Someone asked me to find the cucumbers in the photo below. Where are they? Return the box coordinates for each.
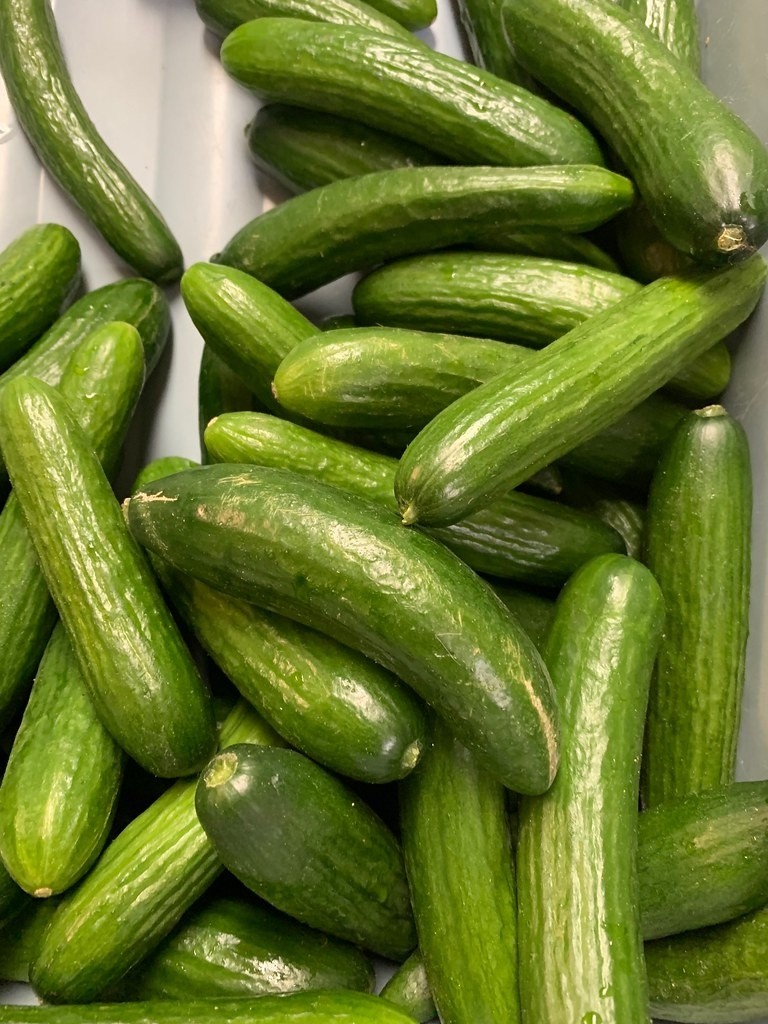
[0,0,768,1024]
